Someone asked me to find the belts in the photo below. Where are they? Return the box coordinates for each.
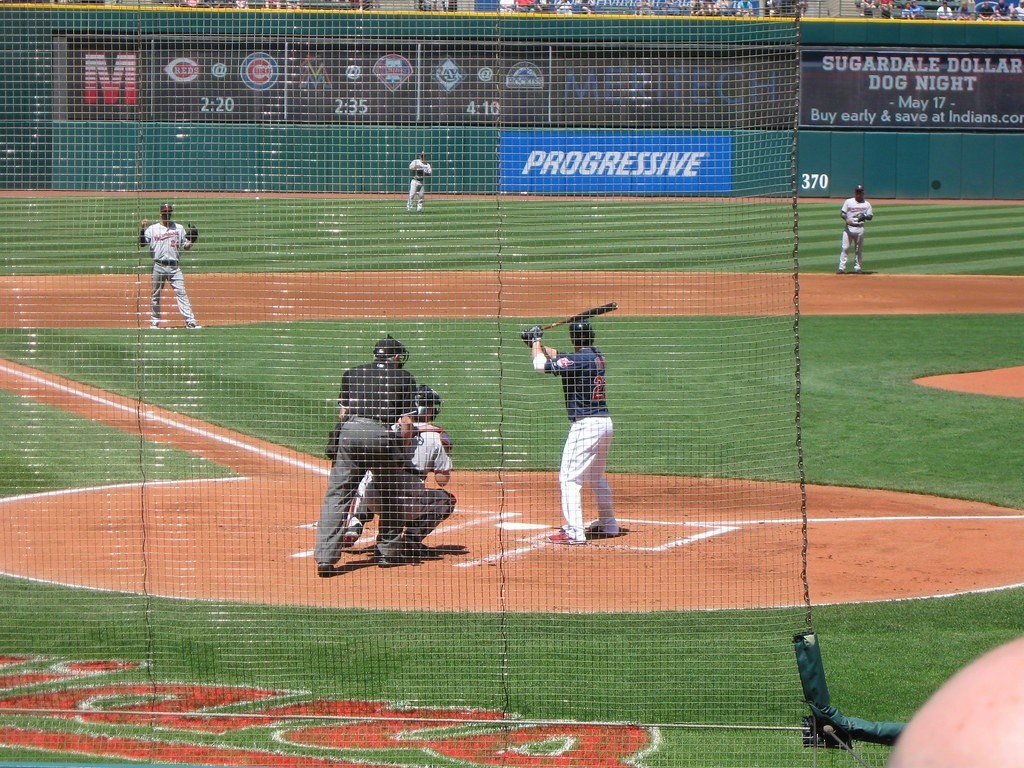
[848,224,859,227]
[348,416,391,428]
[155,260,178,266]
[405,467,423,479]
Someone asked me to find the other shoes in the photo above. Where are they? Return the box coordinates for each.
[378,556,405,568]
[318,561,336,578]
[858,270,861,273]
[549,534,571,545]
[342,524,362,548]
[186,324,202,330]
[588,525,600,533]
[150,325,157,329]
[401,531,431,557]
[836,269,843,274]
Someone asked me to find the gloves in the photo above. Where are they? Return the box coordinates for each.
[525,327,544,347]
[186,224,197,243]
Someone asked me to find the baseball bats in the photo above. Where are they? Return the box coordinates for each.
[521,302,618,338]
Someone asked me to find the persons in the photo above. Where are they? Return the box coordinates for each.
[689,0,755,16]
[419,0,458,12]
[139,203,204,329]
[662,0,681,15]
[766,0,809,18]
[312,334,419,577]
[936,0,1024,21]
[837,185,874,273]
[636,0,655,15]
[175,0,301,10]
[859,0,928,20]
[496,0,597,14]
[528,319,621,545]
[342,381,453,548]
[406,152,432,214]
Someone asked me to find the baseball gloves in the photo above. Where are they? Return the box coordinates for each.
[185,223,198,243]
[854,213,865,222]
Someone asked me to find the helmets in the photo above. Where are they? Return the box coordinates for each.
[415,384,442,419]
[570,320,595,348]
[855,185,864,190]
[420,151,427,156]
[161,203,173,212]
[374,334,409,369]
